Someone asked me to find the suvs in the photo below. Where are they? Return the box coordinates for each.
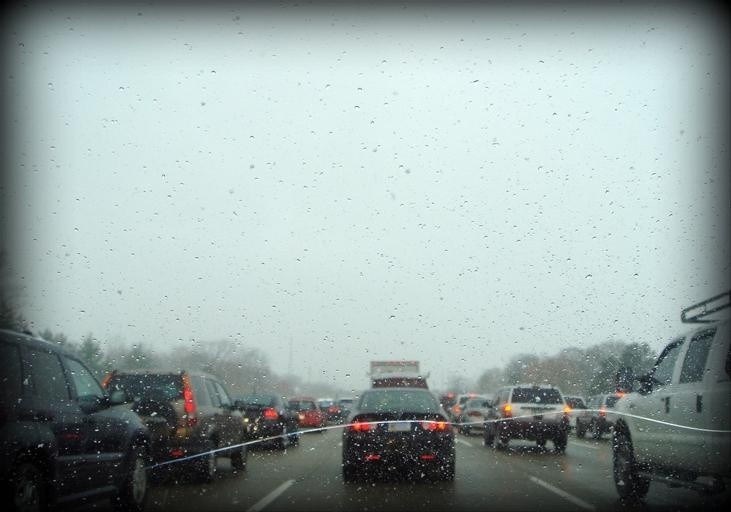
[97,364,253,483]
[0,322,156,510]
[483,382,573,452]
[575,391,629,438]
[608,285,731,500]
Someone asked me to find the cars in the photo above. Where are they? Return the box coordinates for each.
[340,383,459,481]
[287,392,499,435]
[227,389,302,450]
[561,391,586,428]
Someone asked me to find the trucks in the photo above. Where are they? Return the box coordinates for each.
[369,359,428,388]
